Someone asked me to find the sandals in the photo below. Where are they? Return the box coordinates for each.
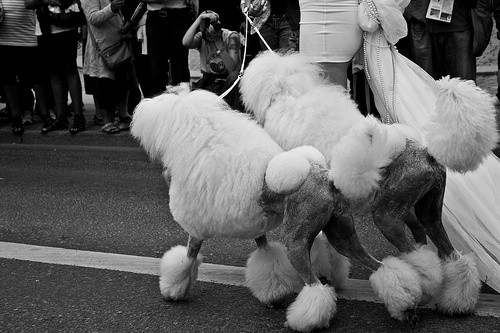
[42,115,69,132]
[70,115,85,134]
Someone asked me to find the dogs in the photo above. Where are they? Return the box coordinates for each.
[239,47,499,319]
[129,83,422,331]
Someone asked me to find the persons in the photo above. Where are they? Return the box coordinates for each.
[0,0,500,137]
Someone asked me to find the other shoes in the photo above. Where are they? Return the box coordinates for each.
[22,110,34,126]
[12,117,24,136]
[101,118,130,133]
[93,114,104,125]
[115,111,132,122]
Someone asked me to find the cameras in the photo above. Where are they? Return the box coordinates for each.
[204,18,221,33]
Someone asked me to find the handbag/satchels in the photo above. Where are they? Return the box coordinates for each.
[101,39,133,70]
